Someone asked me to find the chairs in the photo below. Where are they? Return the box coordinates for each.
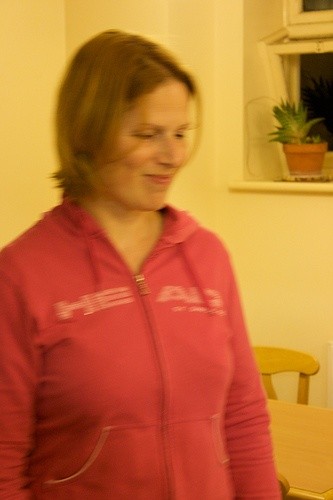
[249,346,320,405]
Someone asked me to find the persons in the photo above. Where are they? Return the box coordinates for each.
[0,29,283,500]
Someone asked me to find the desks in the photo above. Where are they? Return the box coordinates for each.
[266,400,333,500]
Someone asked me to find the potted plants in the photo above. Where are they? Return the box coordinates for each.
[268,98,330,182]
